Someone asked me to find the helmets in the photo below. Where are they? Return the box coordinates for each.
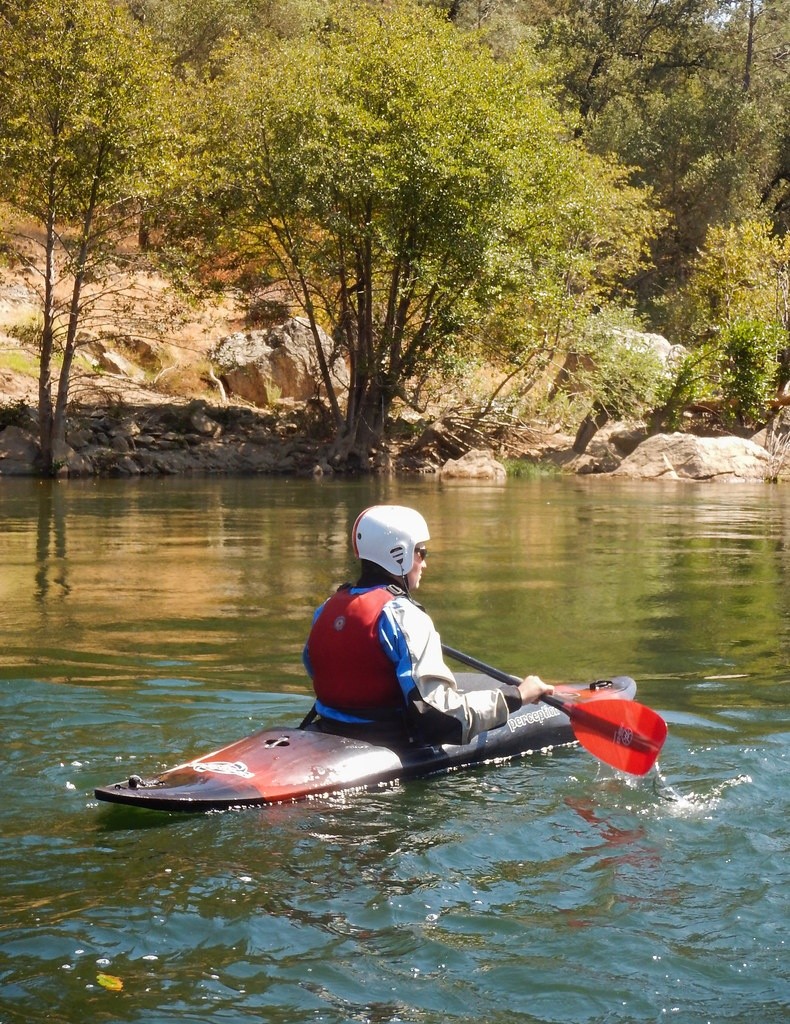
[352,505,430,575]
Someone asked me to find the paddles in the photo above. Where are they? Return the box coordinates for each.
[438,642,670,779]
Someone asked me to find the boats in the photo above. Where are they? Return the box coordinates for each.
[95,678,637,817]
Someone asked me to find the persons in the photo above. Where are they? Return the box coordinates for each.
[303,504,555,752]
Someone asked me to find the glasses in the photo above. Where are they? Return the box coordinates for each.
[414,547,428,562]
